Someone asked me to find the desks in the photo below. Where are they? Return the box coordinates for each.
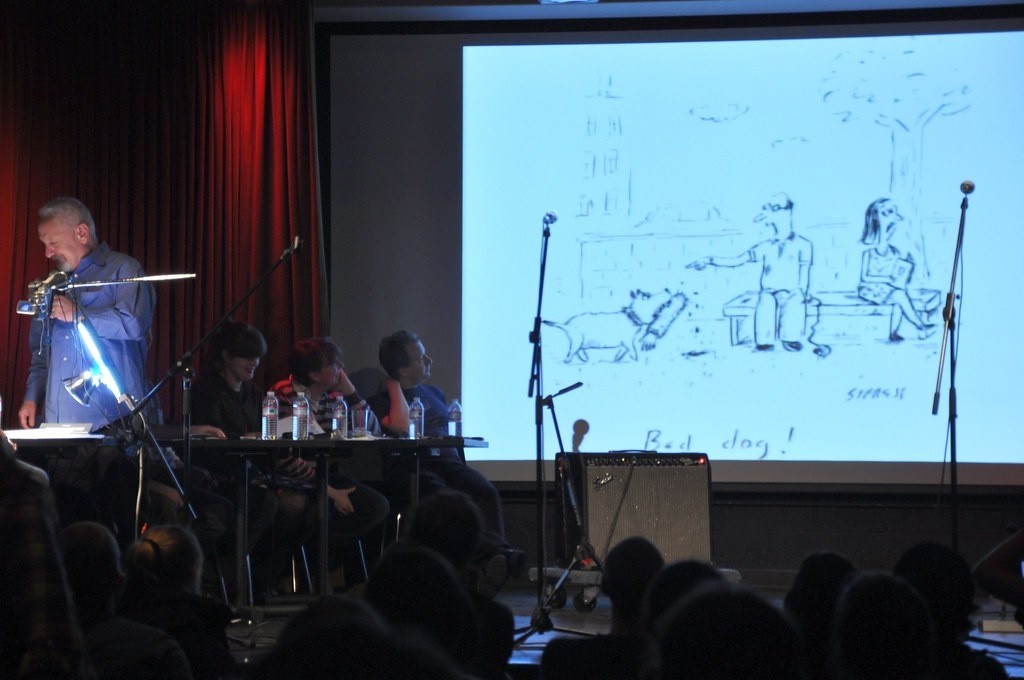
[149,438,489,616]
[9,435,120,527]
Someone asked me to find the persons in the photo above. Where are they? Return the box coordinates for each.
[367,328,529,602]
[17,196,157,567]
[267,334,391,598]
[0,429,517,680]
[540,536,1009,680]
[102,326,237,599]
[991,525,1024,626]
[187,320,314,605]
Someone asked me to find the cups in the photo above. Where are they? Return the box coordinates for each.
[352,410,367,436]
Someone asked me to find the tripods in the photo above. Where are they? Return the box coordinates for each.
[512,223,600,648]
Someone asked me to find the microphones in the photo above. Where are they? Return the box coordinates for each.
[543,210,557,223]
[961,180,975,194]
[47,270,66,297]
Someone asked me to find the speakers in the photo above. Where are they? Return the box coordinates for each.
[555,453,712,565]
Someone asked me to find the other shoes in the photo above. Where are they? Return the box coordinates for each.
[497,547,528,579]
[481,554,507,600]
[249,583,297,603]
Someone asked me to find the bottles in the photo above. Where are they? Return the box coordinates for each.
[261,391,278,439]
[449,398,462,437]
[332,396,347,438]
[409,397,424,440]
[293,392,309,441]
